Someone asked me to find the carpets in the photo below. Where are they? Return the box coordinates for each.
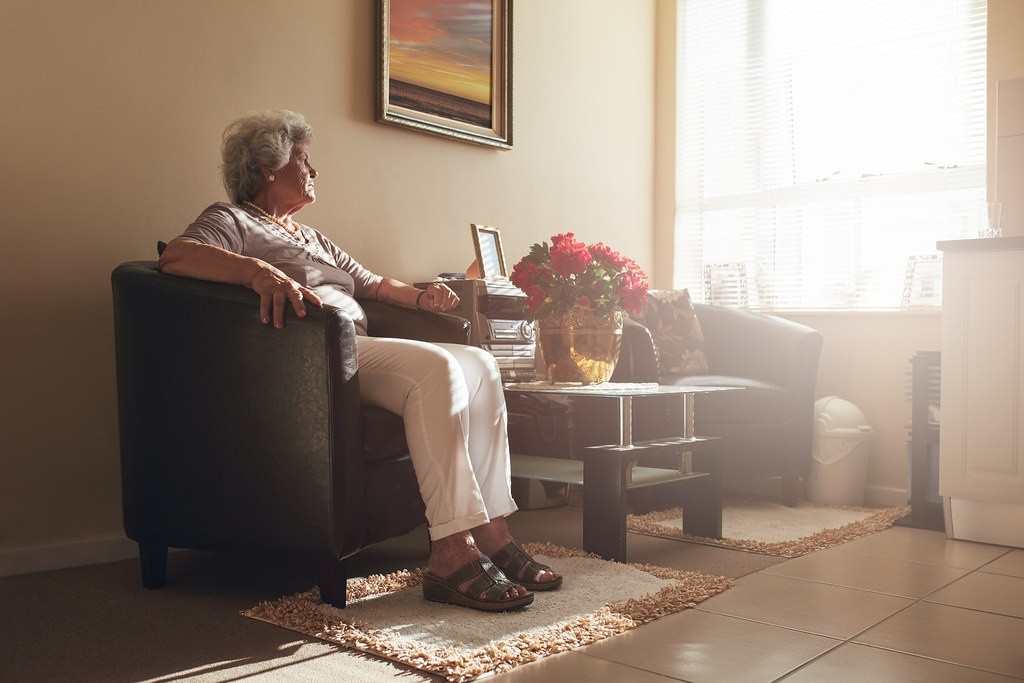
[624,502,912,561]
[240,539,734,683]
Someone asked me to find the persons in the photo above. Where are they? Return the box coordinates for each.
[156,107,565,611]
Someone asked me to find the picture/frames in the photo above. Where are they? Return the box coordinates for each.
[470,224,508,279]
[374,0,514,152]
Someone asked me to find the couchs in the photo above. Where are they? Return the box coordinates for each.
[541,297,824,516]
[110,258,473,608]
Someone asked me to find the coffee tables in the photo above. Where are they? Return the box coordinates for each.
[499,381,745,563]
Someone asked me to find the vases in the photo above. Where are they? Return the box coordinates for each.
[535,311,622,386]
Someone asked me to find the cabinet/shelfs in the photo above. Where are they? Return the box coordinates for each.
[413,278,571,510]
[937,235,1024,549]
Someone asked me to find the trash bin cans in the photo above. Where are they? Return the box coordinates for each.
[806,395,874,507]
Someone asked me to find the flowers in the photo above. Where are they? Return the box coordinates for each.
[510,234,650,313]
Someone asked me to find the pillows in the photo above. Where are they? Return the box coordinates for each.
[630,288,709,376]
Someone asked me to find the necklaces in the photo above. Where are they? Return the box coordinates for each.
[242,199,302,241]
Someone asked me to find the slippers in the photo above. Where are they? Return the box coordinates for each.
[420,558,533,612]
[491,541,562,591]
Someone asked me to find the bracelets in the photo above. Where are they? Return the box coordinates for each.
[416,290,426,311]
